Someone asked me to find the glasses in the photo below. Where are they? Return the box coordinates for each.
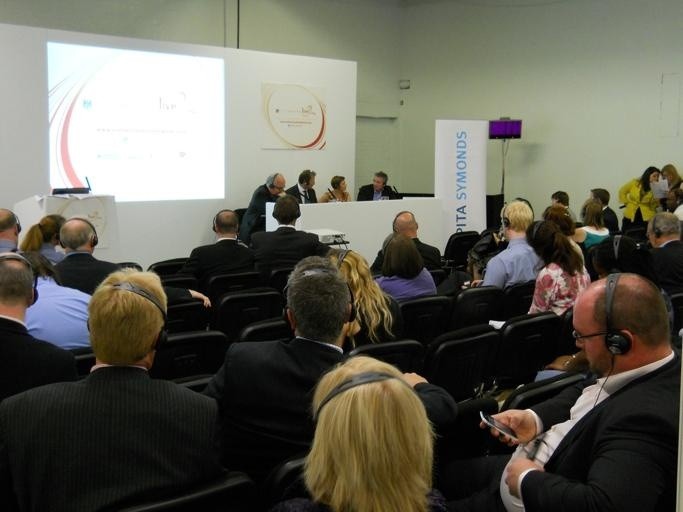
[572,330,606,340]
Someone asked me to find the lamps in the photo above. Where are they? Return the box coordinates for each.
[488,118,521,206]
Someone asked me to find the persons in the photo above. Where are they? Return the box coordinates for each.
[18,250,93,351]
[373,232,437,301]
[665,189,683,221]
[284,170,318,204]
[570,199,609,250]
[268,355,448,512]
[51,218,121,296]
[436,199,545,297]
[544,235,674,371]
[658,164,683,213]
[0,251,80,403]
[238,173,286,248]
[248,196,332,270]
[589,189,619,232]
[645,212,683,296]
[19,215,67,266]
[369,211,442,276]
[0,209,24,255]
[318,175,351,203]
[428,273,682,511]
[0,267,220,512]
[198,256,458,508]
[542,205,584,267]
[525,220,591,317]
[356,171,402,201]
[189,210,255,291]
[551,191,576,224]
[324,248,401,361]
[618,166,660,235]
[450,198,534,282]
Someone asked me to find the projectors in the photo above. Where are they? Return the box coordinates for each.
[303,228,345,245]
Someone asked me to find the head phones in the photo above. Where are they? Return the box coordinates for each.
[303,170,313,186]
[392,210,419,232]
[281,267,358,325]
[269,172,279,189]
[58,218,100,249]
[606,234,623,273]
[0,251,39,308]
[604,273,631,356]
[85,281,170,352]
[212,209,241,235]
[653,214,662,239]
[310,370,438,429]
[530,221,547,247]
[500,198,535,227]
[15,212,22,235]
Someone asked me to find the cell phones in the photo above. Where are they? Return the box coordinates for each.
[479,410,519,442]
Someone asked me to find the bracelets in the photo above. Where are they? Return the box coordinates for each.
[559,354,578,368]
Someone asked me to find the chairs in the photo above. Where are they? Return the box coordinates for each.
[582,242,606,280]
[217,289,283,331]
[429,326,502,397]
[500,313,560,370]
[235,315,292,342]
[207,274,262,296]
[166,302,214,333]
[266,269,294,291]
[426,269,446,288]
[167,330,227,371]
[123,475,257,512]
[162,276,201,296]
[263,452,310,512]
[503,281,535,315]
[455,395,499,420]
[348,341,426,374]
[149,258,190,276]
[397,296,453,339]
[625,228,649,244]
[234,208,249,232]
[446,231,479,263]
[502,373,585,413]
[452,285,502,329]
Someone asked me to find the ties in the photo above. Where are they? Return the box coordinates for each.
[304,190,309,203]
